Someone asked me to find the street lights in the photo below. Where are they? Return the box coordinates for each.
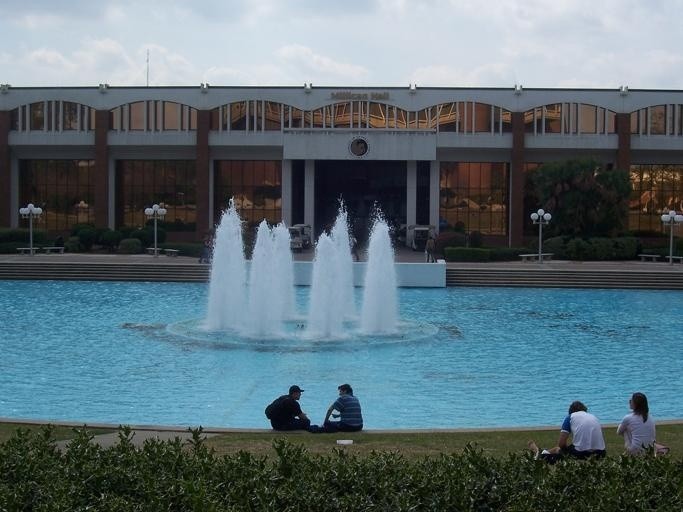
[19,203,43,257]
[530,208,552,264]
[661,210,683,266]
[144,204,168,259]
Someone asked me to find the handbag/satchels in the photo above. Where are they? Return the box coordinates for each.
[266,399,282,419]
[541,453,559,465]
[654,443,670,456]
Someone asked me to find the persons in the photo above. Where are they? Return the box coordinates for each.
[528,400,608,461]
[615,392,656,456]
[349,231,360,261]
[270,385,311,431]
[198,236,213,264]
[321,383,364,432]
[425,236,436,263]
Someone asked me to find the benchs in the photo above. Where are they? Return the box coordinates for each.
[145,247,180,257]
[637,252,683,265]
[517,252,556,264]
[16,247,66,255]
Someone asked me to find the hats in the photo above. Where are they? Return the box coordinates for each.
[289,385,304,393]
